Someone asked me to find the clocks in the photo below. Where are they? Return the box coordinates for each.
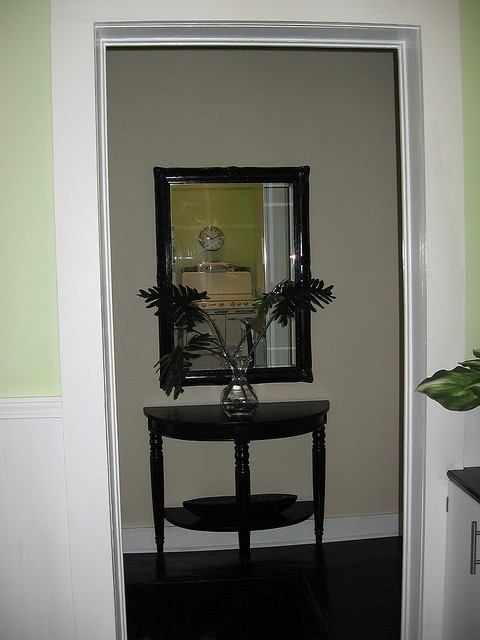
[199,226,225,251]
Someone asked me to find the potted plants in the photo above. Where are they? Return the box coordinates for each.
[137,273,336,421]
[169,275,299,370]
[414,347,479,412]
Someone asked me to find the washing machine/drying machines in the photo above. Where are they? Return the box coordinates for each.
[181,259,256,372]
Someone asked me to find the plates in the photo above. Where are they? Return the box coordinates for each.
[183,494,298,518]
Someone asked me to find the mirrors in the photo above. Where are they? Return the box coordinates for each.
[154,165,314,388]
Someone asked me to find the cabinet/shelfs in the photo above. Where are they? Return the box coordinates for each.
[140,399,332,573]
[444,465,480,640]
